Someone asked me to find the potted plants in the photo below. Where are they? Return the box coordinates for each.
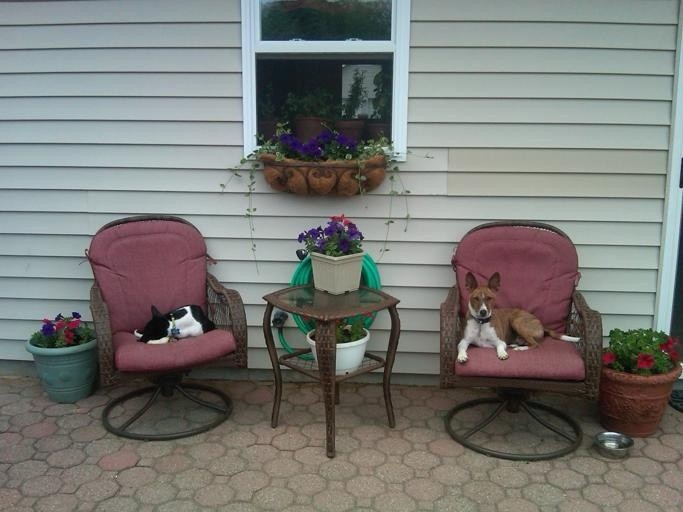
[256,85,284,140]
[289,79,328,140]
[370,76,394,144]
[337,70,363,144]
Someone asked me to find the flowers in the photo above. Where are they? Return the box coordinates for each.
[309,315,375,344]
[35,308,87,342]
[599,324,681,376]
[25,310,95,351]
[293,214,366,261]
[296,214,365,253]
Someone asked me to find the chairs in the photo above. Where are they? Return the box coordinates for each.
[439,219,603,461]
[82,211,249,441]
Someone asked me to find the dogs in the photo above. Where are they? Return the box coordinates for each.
[457,270,581,367]
[133,301,217,347]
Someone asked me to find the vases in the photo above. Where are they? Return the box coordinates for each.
[306,323,370,373]
[22,327,97,403]
[304,249,368,296]
[591,359,682,437]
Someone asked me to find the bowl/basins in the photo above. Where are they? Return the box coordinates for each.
[596,432,634,459]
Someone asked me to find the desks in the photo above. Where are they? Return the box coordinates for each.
[271,283,403,458]
